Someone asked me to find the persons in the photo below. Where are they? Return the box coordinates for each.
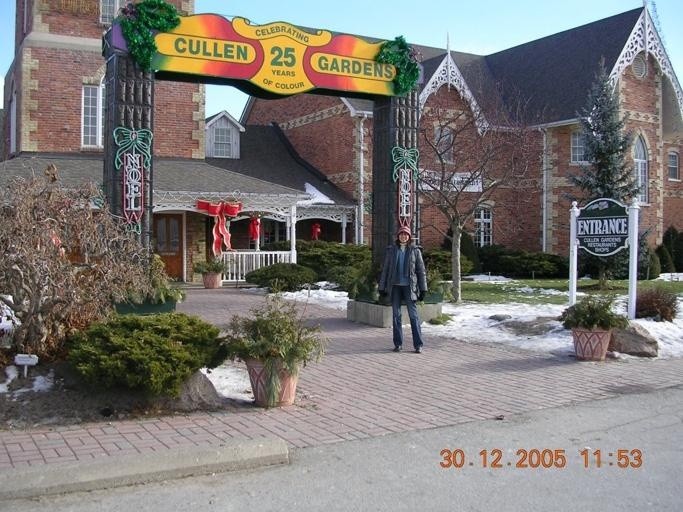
[377,225,430,354]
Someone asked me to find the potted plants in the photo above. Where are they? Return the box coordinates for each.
[222,278,332,407]
[191,254,231,288]
[556,293,632,361]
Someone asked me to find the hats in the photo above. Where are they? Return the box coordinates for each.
[396,227,411,237]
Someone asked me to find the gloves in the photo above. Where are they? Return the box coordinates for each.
[379,290,387,297]
[418,291,425,301]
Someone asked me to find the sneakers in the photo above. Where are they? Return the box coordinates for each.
[394,345,402,352]
[415,347,422,353]
